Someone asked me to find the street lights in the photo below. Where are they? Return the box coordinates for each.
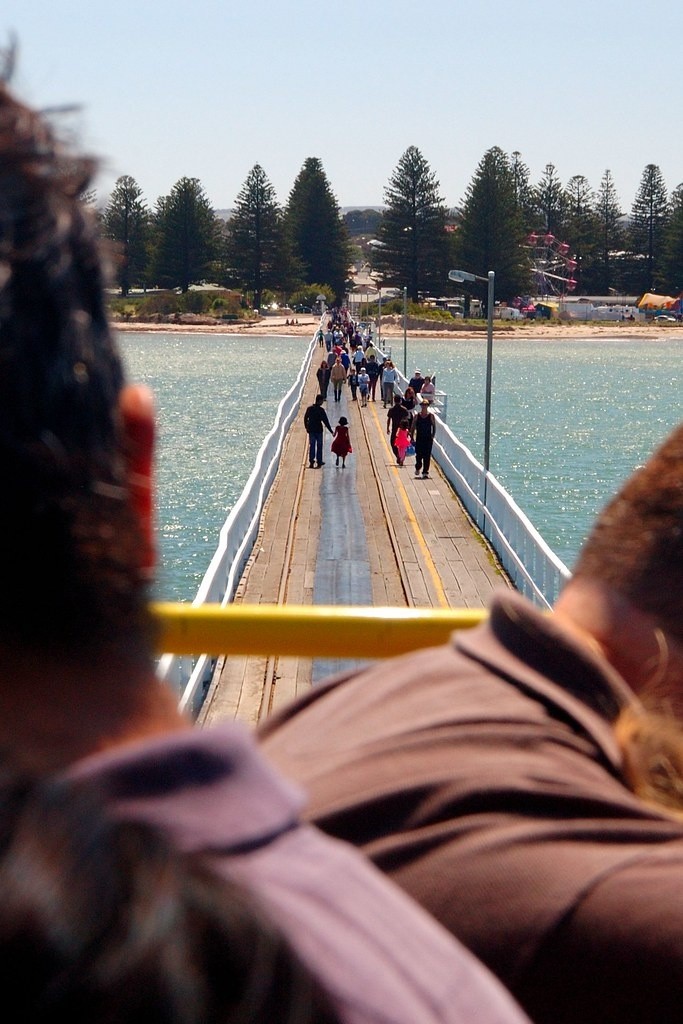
[447,268,495,472]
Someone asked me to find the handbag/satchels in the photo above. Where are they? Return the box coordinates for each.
[353,358,355,365]
[406,445,415,457]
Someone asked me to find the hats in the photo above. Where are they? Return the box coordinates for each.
[335,357,342,362]
[415,368,421,373]
[361,367,366,372]
[419,399,429,406]
[340,350,346,354]
[338,417,348,425]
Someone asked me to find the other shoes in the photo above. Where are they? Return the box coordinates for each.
[372,398,376,402]
[317,461,325,465]
[361,403,367,408]
[424,472,429,478]
[336,460,339,466]
[310,459,314,463]
[415,470,420,475]
[342,464,346,469]
[367,395,369,400]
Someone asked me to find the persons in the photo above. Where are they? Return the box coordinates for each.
[0,57,532,1024]
[253,428,683,1024]
[304,306,437,478]
